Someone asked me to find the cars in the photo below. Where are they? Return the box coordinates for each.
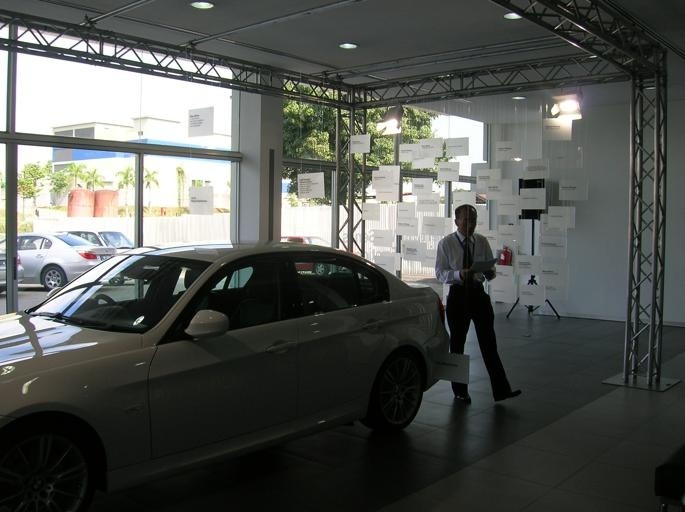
[1,242,453,510]
[281,235,358,276]
[0,228,136,294]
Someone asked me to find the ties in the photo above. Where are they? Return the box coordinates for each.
[462,238,475,282]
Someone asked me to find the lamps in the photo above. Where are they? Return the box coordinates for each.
[558,85,584,121]
[377,105,404,134]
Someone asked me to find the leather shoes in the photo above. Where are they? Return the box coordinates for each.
[494,390,521,401]
[456,394,472,403]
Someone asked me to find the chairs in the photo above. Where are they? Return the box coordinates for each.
[324,271,359,307]
[145,269,216,336]
[229,278,277,330]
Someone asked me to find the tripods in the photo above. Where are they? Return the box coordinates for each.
[503,220,561,320]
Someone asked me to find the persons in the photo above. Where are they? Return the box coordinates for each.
[435,205,522,404]
